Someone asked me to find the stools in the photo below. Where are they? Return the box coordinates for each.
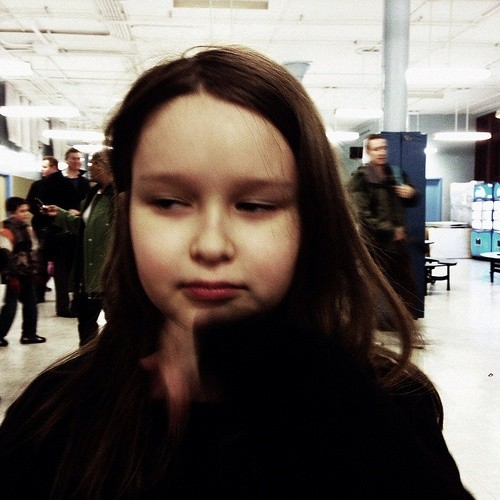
[480,252,500,283]
[425,256,457,291]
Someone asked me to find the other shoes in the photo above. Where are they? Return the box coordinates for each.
[45,286,51,292]
[20,335,46,344]
[0,337,9,348]
[56,309,77,318]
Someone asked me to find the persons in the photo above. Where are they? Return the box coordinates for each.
[0,47,475,500]
[25,149,115,347]
[0,197,46,347]
[349,135,430,348]
